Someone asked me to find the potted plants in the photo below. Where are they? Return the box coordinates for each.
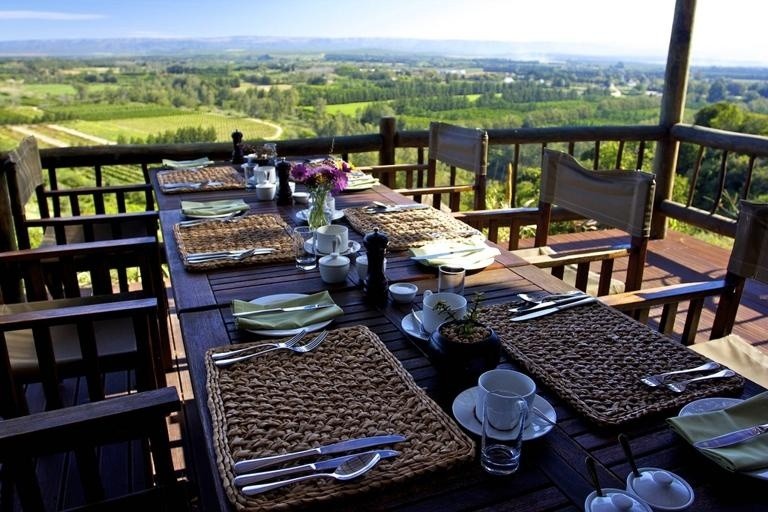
[426,291,500,378]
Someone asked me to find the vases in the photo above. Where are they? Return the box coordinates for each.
[307,195,334,246]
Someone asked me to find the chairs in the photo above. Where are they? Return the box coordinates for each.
[1,297,185,512]
[452,149,655,296]
[589,200,768,391]
[3,236,175,391]
[1,134,160,297]
[355,122,490,214]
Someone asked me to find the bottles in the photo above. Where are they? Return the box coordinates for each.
[363,227,389,296]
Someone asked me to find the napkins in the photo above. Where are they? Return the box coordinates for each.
[668,392,768,471]
[230,288,344,331]
[176,198,251,220]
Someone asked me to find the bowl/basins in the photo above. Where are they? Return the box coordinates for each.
[388,283,417,303]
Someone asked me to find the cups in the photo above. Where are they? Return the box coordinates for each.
[583,487,654,512]
[478,391,524,477]
[315,252,389,282]
[476,368,538,430]
[625,466,697,511]
[423,263,467,333]
[308,197,335,216]
[264,143,278,162]
[316,224,348,250]
[292,226,318,272]
[240,161,336,215]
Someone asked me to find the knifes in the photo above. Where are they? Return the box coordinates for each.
[235,433,406,472]
[235,302,333,320]
[187,248,277,258]
[365,203,428,212]
[232,448,401,488]
[507,293,596,323]
[692,423,768,453]
[409,245,488,261]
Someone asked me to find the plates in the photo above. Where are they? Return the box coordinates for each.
[244,293,335,336]
[448,382,561,438]
[294,209,348,223]
[399,310,430,342]
[342,182,374,191]
[675,396,743,420]
[302,237,360,255]
[413,246,501,271]
[183,211,244,219]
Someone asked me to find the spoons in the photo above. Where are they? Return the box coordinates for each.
[240,450,381,499]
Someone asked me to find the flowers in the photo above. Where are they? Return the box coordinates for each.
[287,158,354,195]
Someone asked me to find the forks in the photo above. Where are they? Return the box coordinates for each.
[206,326,330,365]
[187,249,256,265]
[631,361,736,392]
